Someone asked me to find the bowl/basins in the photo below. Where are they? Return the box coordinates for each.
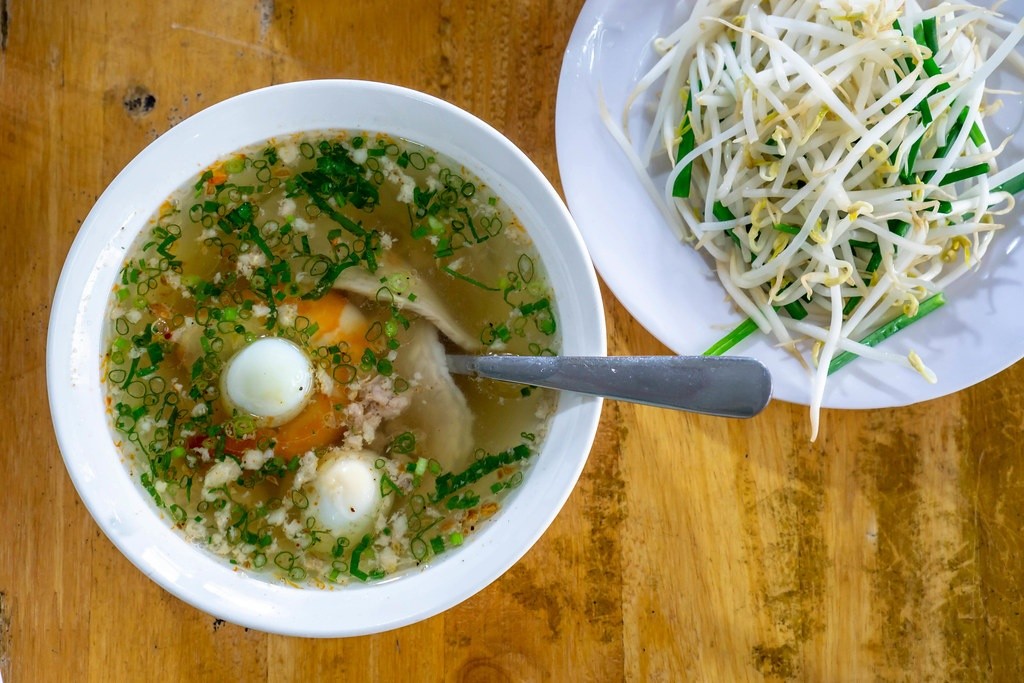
[42,78,614,640]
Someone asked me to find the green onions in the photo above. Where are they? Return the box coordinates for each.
[107,135,558,587]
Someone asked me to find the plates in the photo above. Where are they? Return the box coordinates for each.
[552,0,1024,409]
